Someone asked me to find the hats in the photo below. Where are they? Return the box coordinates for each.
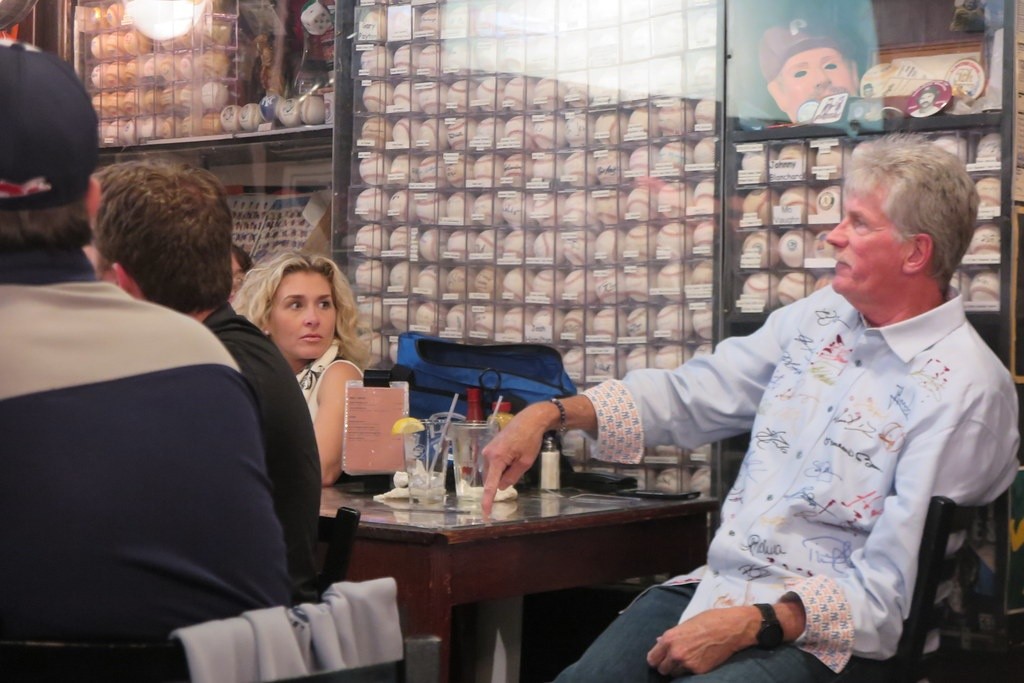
[0,42,98,210]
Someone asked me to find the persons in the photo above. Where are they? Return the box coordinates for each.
[0,35,292,643]
[480,133,1021,683]
[232,247,373,486]
[229,242,253,301]
[94,159,321,606]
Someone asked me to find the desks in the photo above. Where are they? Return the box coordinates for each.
[309,489,721,683]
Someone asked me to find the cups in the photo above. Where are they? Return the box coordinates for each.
[447,422,499,509]
[402,419,452,506]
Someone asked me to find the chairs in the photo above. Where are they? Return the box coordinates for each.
[883,496,986,683]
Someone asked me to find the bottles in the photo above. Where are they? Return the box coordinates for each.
[486,402,516,432]
[539,437,561,493]
[459,388,488,489]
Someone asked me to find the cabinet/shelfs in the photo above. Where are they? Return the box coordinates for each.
[63,1,1023,626]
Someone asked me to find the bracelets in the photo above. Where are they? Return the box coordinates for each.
[549,398,566,432]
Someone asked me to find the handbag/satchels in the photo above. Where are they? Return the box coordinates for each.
[395,332,577,475]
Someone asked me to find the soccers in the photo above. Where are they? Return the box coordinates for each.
[350,1,710,499]
[87,5,336,140]
[733,131,1001,306]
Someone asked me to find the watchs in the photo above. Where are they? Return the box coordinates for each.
[754,603,783,649]
